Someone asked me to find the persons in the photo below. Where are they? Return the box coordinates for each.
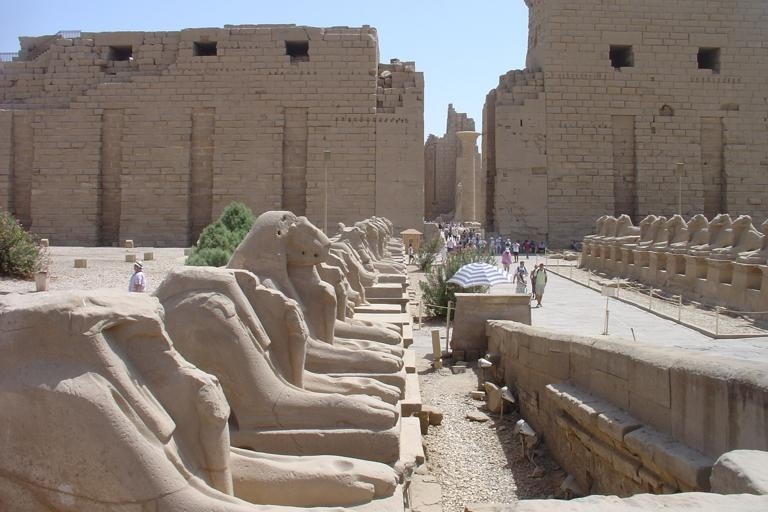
[129,260,146,292]
[407,218,580,308]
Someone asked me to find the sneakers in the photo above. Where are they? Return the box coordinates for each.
[536,304,543,307]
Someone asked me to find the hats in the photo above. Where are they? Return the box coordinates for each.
[538,264,545,266]
[133,261,143,268]
